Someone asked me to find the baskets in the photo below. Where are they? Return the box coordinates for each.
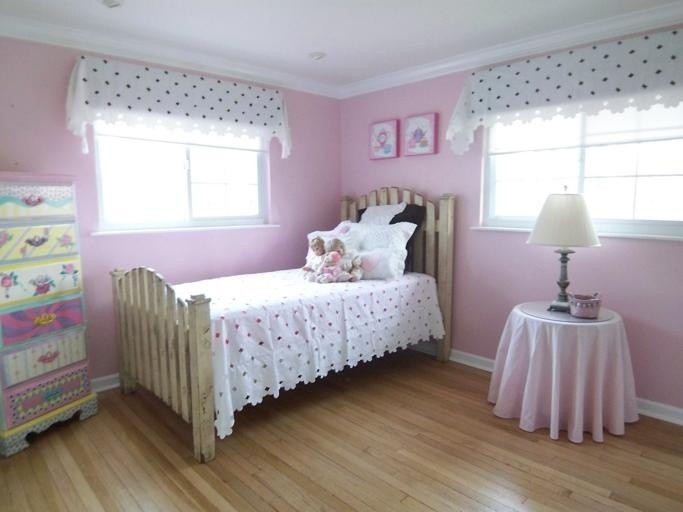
[566,292,602,319]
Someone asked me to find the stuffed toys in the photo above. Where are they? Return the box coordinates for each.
[316,251,350,283]
[301,237,325,281]
[314,239,363,281]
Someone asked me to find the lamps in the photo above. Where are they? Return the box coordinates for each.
[526,190,600,314]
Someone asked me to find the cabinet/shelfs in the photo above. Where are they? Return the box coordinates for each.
[0,171,100,456]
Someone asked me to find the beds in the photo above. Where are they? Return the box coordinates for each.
[108,189,457,464]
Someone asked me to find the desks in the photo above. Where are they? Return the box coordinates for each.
[483,301,641,447]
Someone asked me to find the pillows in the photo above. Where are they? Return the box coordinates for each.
[334,220,416,281]
[355,202,424,248]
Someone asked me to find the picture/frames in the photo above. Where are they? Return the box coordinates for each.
[366,119,399,161]
[400,112,440,157]
